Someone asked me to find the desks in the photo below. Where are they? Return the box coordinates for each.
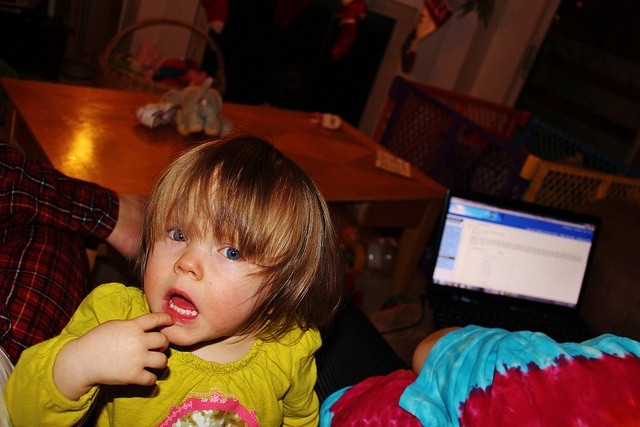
[1,72,451,320]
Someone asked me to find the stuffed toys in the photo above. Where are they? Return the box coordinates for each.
[133,76,234,137]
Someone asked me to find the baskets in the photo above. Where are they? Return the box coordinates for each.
[98,15,226,101]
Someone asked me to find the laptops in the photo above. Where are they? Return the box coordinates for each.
[429,190,602,342]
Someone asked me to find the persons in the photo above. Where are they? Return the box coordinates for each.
[3,133,345,426]
[1,142,150,368]
[314,322,640,426]
